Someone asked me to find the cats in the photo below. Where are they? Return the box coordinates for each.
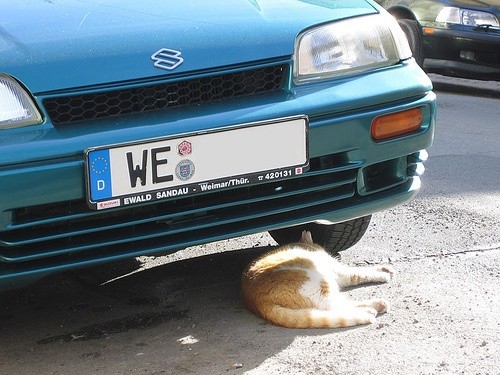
[241,230,394,329]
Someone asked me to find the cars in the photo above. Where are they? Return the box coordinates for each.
[0,0,436,299]
[383,0,500,82]
